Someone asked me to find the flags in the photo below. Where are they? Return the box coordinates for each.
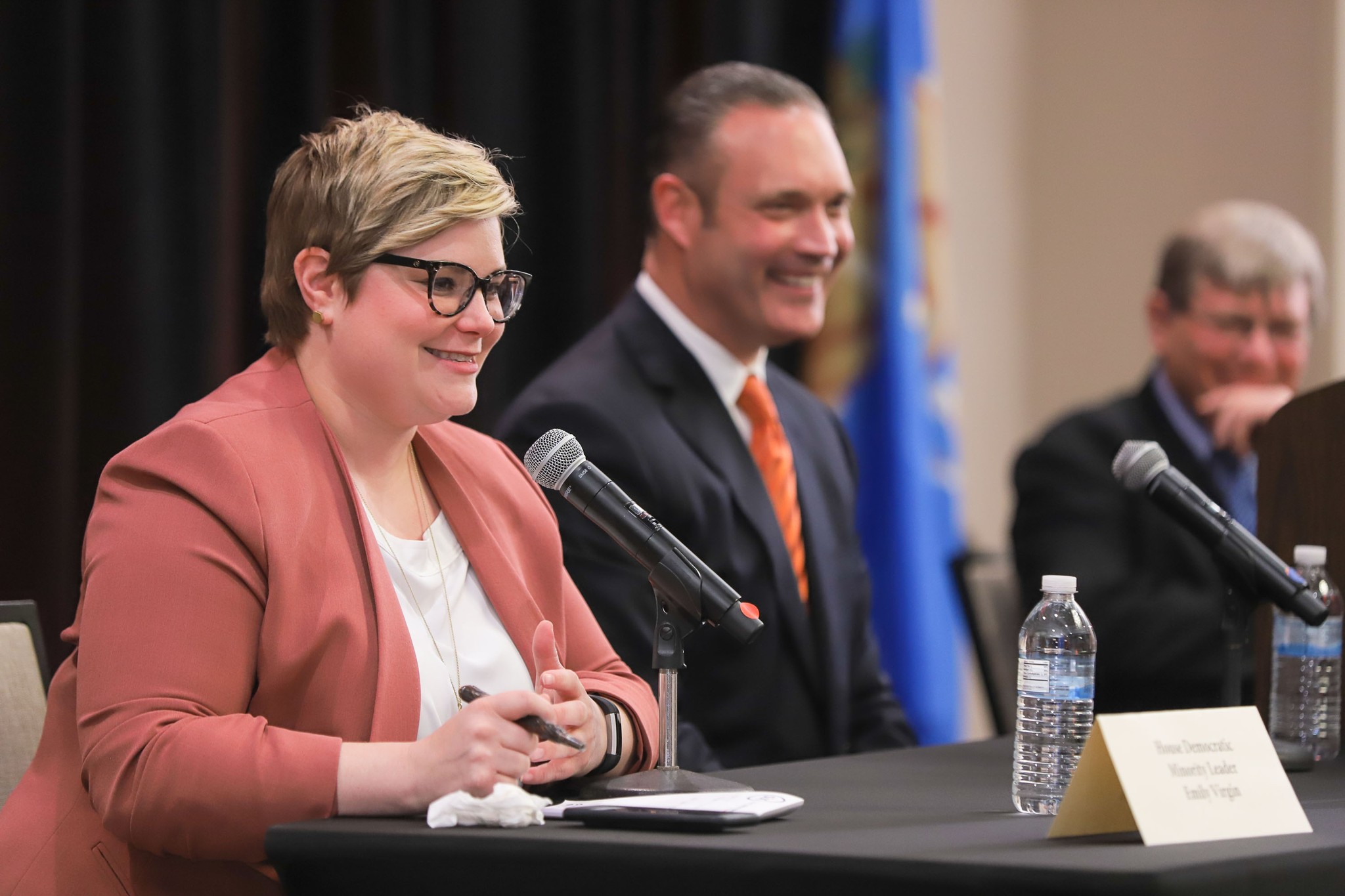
[791,0,1005,743]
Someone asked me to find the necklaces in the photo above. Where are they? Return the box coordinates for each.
[356,441,462,713]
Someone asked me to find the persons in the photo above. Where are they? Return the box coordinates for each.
[1008,195,1324,723]
[0,104,661,896]
[493,59,913,772]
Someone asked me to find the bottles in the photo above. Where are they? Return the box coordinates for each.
[1268,545,1344,763]
[1012,575,1097,814]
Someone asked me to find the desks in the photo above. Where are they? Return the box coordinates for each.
[261,728,1345,896]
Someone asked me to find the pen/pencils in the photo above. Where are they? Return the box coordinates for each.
[457,684,586,750]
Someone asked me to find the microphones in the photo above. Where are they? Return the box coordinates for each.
[1113,439,1329,627]
[523,428,766,644]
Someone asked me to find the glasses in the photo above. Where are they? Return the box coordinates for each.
[366,254,533,323]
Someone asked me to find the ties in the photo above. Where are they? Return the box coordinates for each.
[737,373,807,611]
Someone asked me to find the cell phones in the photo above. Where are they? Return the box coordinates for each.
[563,806,757,831]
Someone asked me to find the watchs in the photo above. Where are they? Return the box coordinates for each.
[581,694,622,778]
